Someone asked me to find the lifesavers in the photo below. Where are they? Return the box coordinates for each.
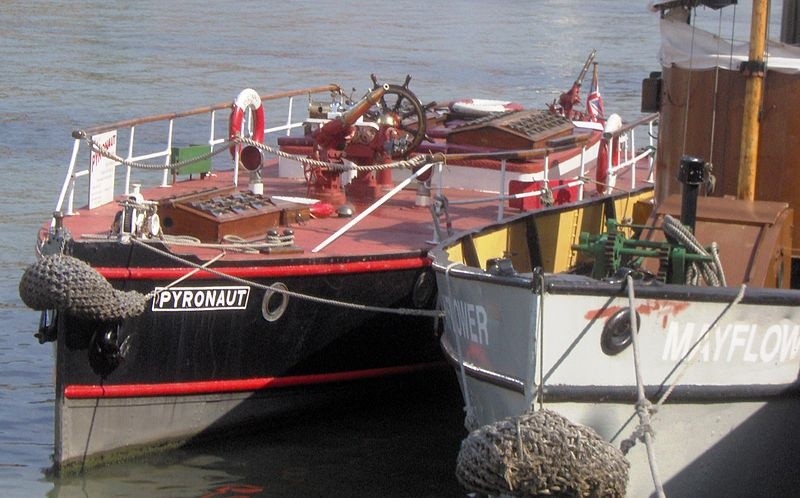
[448,97,521,119]
[229,87,265,165]
[595,112,625,196]
[271,194,335,219]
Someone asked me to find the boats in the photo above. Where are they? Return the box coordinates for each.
[425,2,800,498]
[28,47,657,479]
